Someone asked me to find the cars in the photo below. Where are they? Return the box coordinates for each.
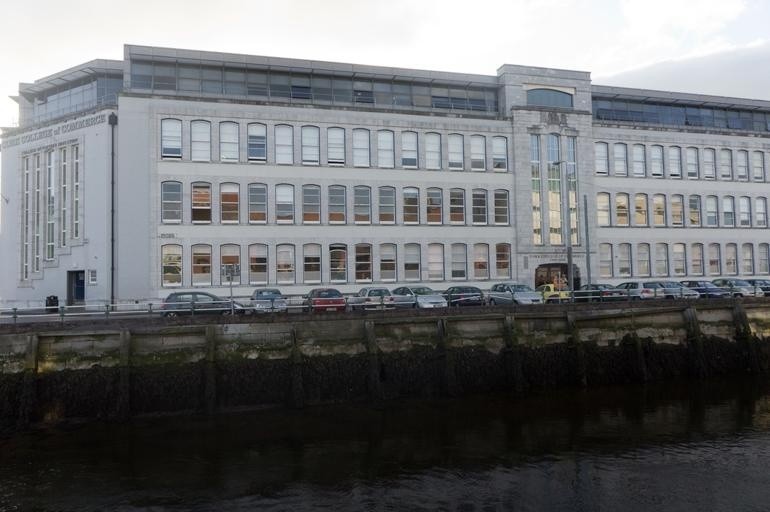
[248,287,289,312]
[351,285,393,311]
[161,265,182,283]
[302,288,346,312]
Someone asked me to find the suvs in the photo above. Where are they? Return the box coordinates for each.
[159,291,246,319]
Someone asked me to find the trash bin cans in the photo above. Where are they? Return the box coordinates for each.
[46,295,58,314]
